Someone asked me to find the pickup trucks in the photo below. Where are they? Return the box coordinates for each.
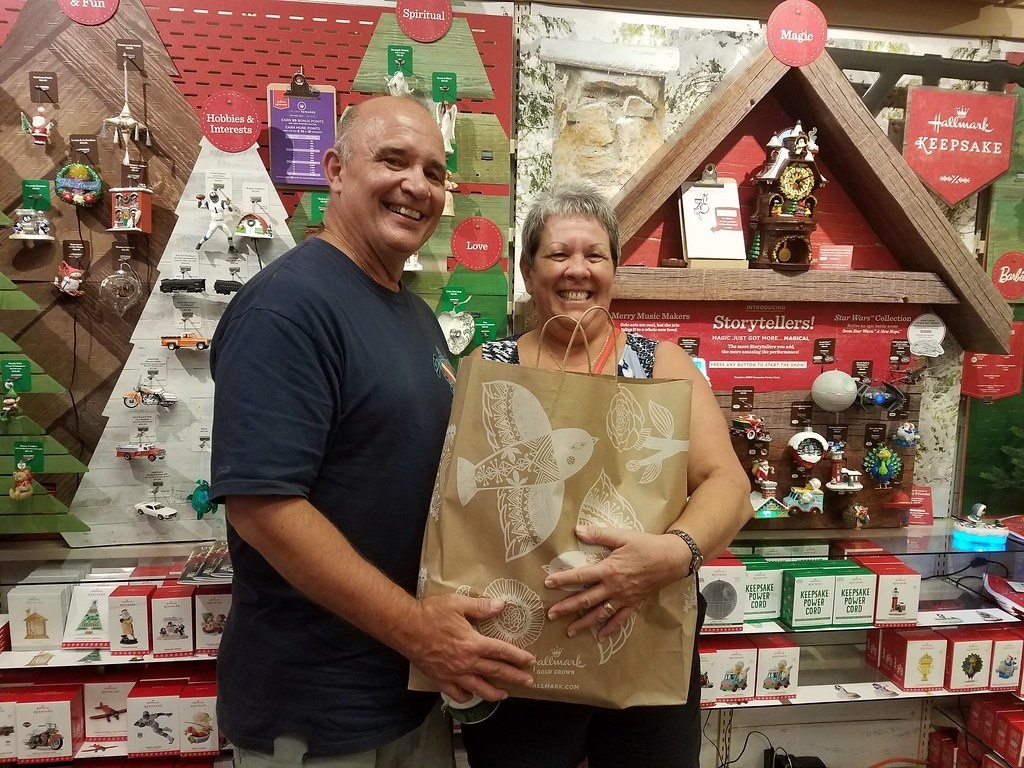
[115,441,166,462]
[161,331,212,351]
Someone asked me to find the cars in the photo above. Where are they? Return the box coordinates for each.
[134,501,178,520]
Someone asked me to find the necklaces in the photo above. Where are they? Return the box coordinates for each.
[539,327,610,371]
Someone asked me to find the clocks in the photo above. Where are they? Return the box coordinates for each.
[750,120,828,271]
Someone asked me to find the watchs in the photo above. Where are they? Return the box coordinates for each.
[666,529,704,578]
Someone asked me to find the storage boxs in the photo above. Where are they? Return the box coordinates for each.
[0,563,238,764]
[698,541,1024,768]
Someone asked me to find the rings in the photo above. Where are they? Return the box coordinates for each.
[602,600,617,616]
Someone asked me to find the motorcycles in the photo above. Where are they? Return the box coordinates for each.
[122,376,177,408]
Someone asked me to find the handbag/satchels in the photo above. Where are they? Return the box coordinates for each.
[405,304,702,711]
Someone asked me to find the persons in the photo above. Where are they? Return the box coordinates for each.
[463,182,753,768]
[133,711,175,746]
[208,92,538,768]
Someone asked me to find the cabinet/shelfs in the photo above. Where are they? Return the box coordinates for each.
[0,579,235,768]
[701,537,1024,711]
